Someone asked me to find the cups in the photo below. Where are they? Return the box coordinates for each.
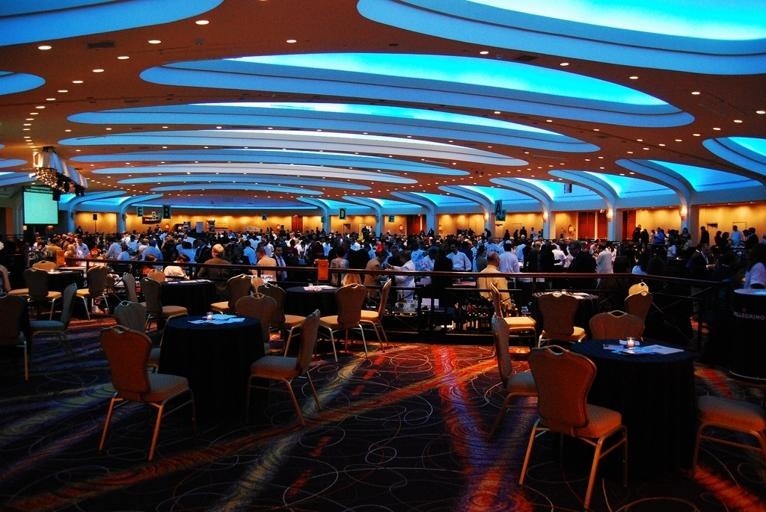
[627,336,637,350]
[205,311,213,320]
[561,288,567,294]
[103,307,109,314]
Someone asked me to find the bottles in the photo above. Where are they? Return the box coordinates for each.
[396,292,418,312]
[745,270,752,291]
[451,300,530,330]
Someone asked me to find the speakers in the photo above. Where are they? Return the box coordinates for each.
[93,214,97,220]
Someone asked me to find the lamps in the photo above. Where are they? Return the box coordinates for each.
[34,146,89,197]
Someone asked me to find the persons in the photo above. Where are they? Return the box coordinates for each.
[1,224,766,318]
